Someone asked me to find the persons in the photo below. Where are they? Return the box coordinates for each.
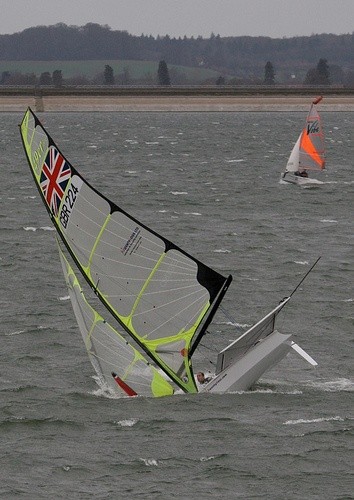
[300,169,309,177]
[196,372,213,384]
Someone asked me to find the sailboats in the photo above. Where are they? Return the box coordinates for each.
[279,95,330,188]
[19,105,319,398]
